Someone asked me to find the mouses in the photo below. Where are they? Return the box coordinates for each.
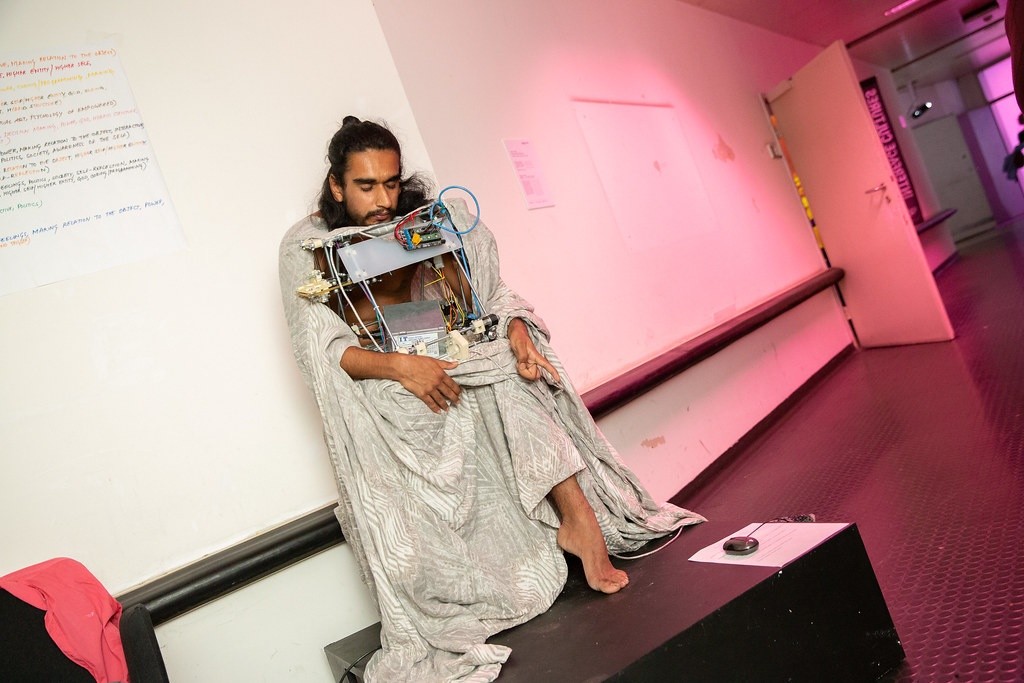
[723,537,759,556]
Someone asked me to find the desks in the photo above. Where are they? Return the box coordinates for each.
[326,523,912,683]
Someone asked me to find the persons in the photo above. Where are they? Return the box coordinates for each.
[275,112,708,683]
